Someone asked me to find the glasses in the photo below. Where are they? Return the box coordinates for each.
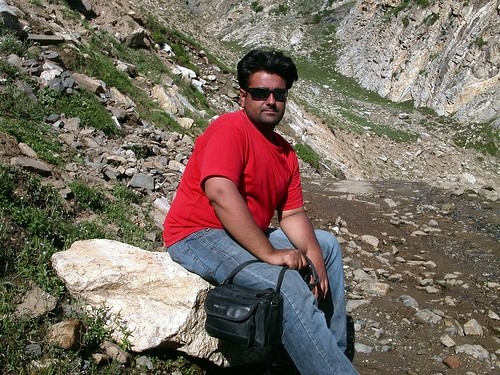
[242,87,289,103]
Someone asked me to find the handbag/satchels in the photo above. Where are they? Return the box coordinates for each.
[203,284,283,354]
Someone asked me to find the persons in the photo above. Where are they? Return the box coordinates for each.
[163,49,358,375]
